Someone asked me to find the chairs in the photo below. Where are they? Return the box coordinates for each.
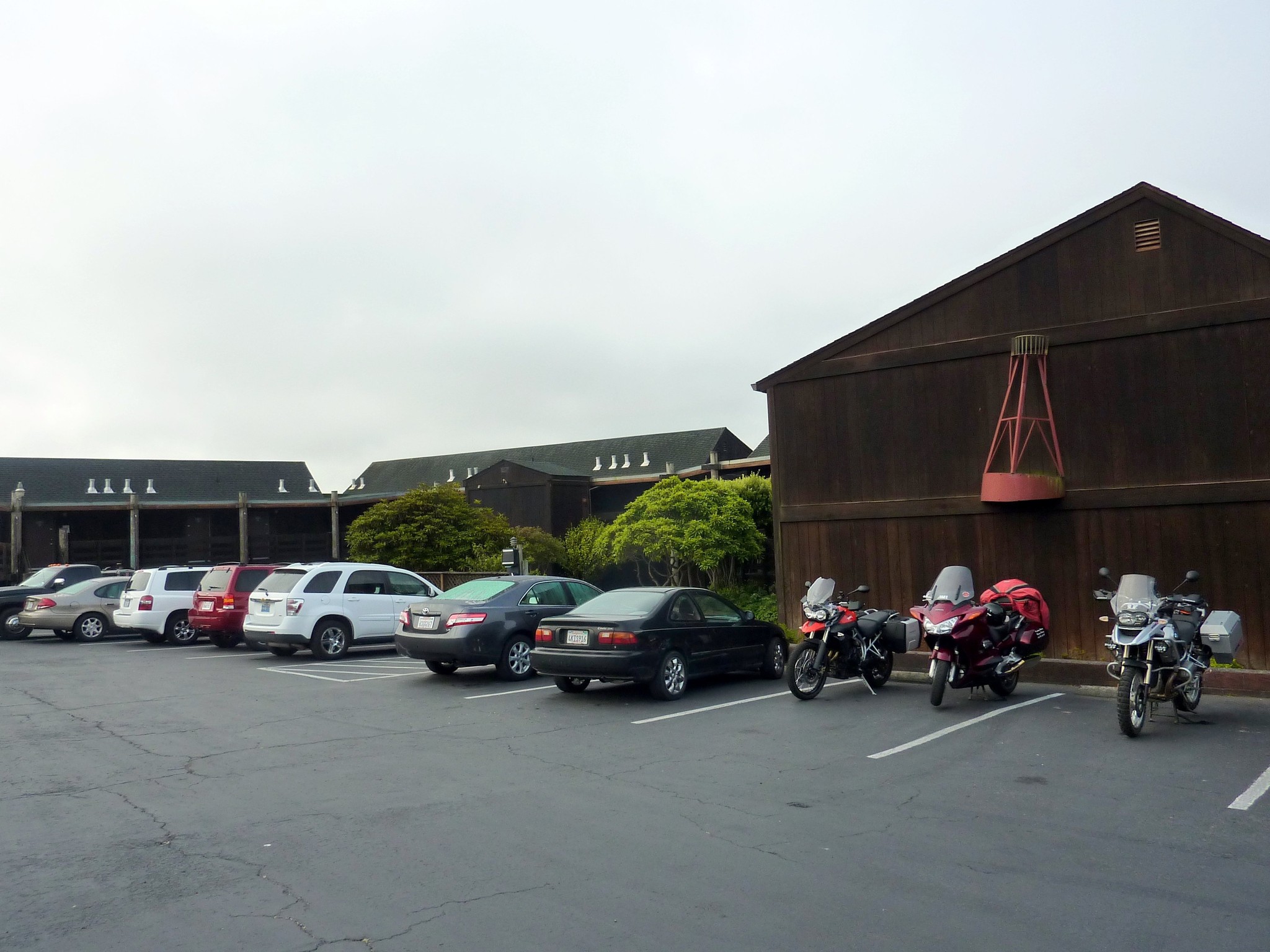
[349,576,366,593]
[679,600,695,621]
[367,575,384,594]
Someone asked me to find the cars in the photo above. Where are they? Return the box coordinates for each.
[528,586,790,699]
[17,575,138,639]
[393,576,608,681]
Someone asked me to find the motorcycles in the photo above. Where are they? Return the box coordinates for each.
[785,577,924,699]
[908,566,1051,705]
[1090,566,1243,733]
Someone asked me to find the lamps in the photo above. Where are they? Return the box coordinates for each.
[510,537,518,550]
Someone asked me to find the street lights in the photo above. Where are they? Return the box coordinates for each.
[9,481,26,585]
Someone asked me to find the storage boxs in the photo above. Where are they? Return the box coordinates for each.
[1200,610,1244,664]
[1015,621,1050,657]
[885,616,923,654]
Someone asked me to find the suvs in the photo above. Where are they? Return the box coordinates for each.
[187,561,293,651]
[244,561,446,660]
[0,564,102,638]
[101,567,137,577]
[112,566,222,646]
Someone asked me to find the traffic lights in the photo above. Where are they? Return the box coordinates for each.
[502,548,516,566]
